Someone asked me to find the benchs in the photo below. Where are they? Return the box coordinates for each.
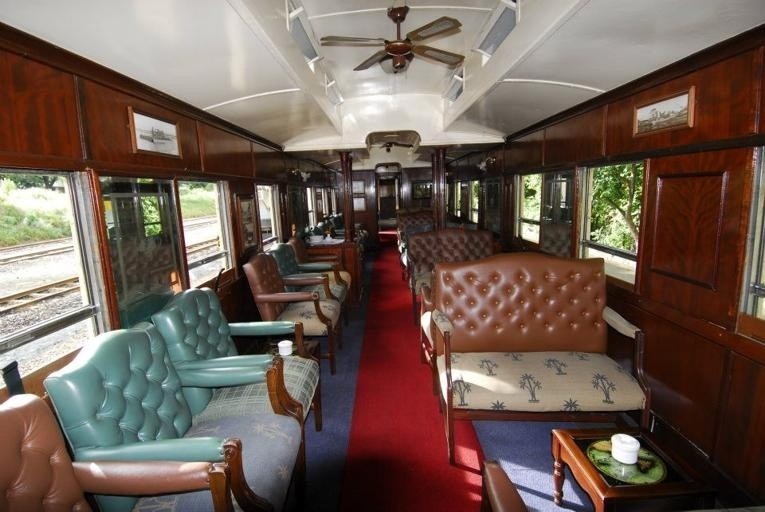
[396,207,499,326]
[419,251,651,466]
[480,462,529,511]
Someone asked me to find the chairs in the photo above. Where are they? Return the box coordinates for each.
[242,236,353,376]
[149,286,323,476]
[307,213,369,264]
[1,321,323,511]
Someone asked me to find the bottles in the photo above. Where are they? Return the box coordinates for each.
[610,432,641,466]
[276,338,294,356]
[325,233,333,243]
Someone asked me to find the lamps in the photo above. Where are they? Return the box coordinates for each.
[474,154,496,174]
[379,54,414,74]
[291,168,313,185]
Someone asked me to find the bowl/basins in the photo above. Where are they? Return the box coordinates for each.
[309,235,322,243]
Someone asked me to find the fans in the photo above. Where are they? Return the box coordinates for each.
[319,6,465,75]
[378,142,413,153]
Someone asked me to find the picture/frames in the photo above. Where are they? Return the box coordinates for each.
[352,179,366,195]
[352,197,368,213]
[231,192,262,262]
[411,179,433,200]
[631,83,698,141]
[126,102,185,163]
